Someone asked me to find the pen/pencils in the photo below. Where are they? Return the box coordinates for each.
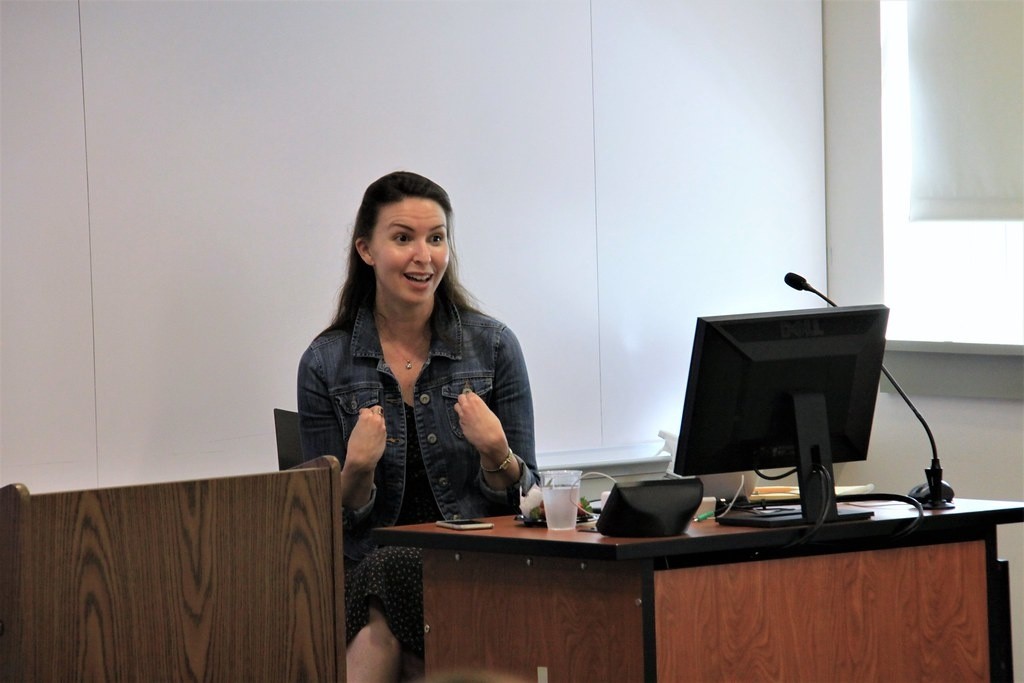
[693,511,715,523]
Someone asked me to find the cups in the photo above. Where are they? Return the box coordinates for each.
[538,470,583,531]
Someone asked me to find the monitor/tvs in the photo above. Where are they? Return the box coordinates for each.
[671,304,890,528]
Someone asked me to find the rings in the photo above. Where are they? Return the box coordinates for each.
[374,407,384,415]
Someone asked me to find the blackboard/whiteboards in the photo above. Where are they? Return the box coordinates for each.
[0,1,830,497]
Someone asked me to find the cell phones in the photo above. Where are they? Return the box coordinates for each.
[436,518,494,530]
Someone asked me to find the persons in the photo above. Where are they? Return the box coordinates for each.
[297,171,541,683]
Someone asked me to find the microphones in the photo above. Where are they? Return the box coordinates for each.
[784,273,956,510]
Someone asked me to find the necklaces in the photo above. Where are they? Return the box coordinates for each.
[381,328,427,370]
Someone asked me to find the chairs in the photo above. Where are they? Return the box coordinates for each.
[0,456,346,683]
[271,404,305,472]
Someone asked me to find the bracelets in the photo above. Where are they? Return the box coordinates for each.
[480,449,512,472]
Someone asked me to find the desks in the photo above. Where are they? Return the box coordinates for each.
[371,501,1024,683]
